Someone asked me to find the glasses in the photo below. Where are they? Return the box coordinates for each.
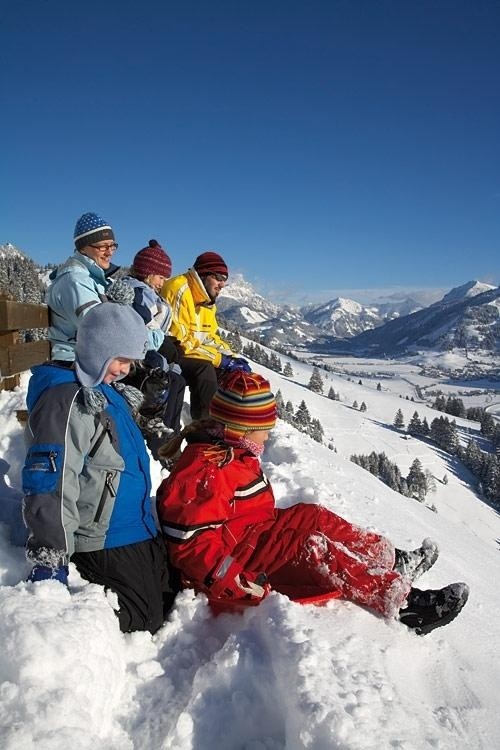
[89,244,118,251]
[211,272,228,283]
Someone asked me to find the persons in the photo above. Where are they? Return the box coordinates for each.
[157,370,469,637]
[47,213,181,440]
[160,251,252,419]
[22,280,171,635]
[122,240,185,471]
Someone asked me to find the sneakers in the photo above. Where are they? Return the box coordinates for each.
[135,412,175,439]
[399,583,470,636]
[160,451,183,472]
[393,537,439,582]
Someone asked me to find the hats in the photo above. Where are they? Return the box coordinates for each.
[74,212,115,252]
[133,240,172,284]
[193,252,229,276]
[204,371,278,466]
[73,301,148,415]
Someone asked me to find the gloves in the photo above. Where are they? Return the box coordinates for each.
[173,340,186,360]
[25,549,69,587]
[143,349,170,379]
[218,353,252,372]
[157,335,178,364]
[203,556,271,606]
